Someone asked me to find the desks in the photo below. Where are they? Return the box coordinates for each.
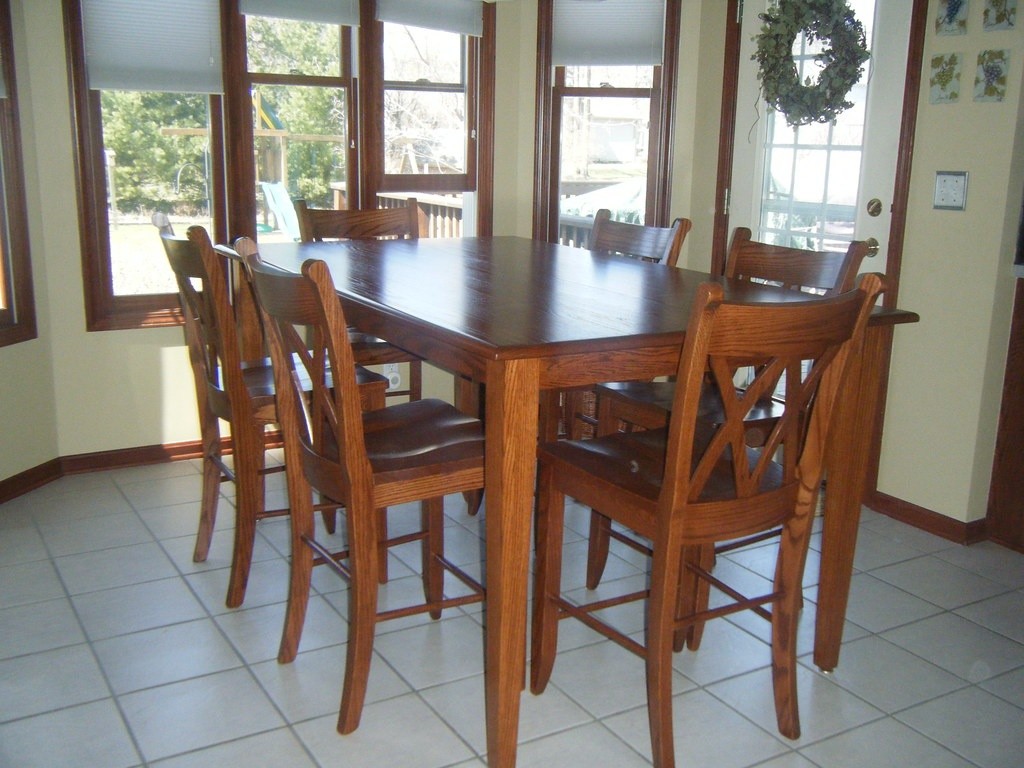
[213,236,921,768]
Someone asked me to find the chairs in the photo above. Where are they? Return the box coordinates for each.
[467,210,692,526]
[585,227,869,652]
[235,237,485,735]
[532,273,889,768]
[294,198,480,500]
[151,211,390,609]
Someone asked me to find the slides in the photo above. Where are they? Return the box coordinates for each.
[259,182,301,240]
